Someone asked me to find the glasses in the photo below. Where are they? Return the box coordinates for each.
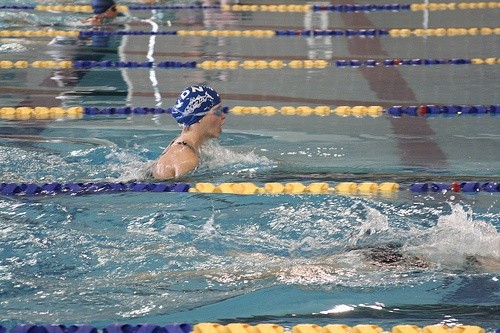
[173,106,224,117]
[110,6,117,12]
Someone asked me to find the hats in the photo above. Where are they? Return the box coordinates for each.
[91,0,115,14]
[171,84,222,126]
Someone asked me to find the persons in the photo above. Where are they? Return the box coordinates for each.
[148,85,229,180]
[77,0,117,30]
[82,220,499,305]
